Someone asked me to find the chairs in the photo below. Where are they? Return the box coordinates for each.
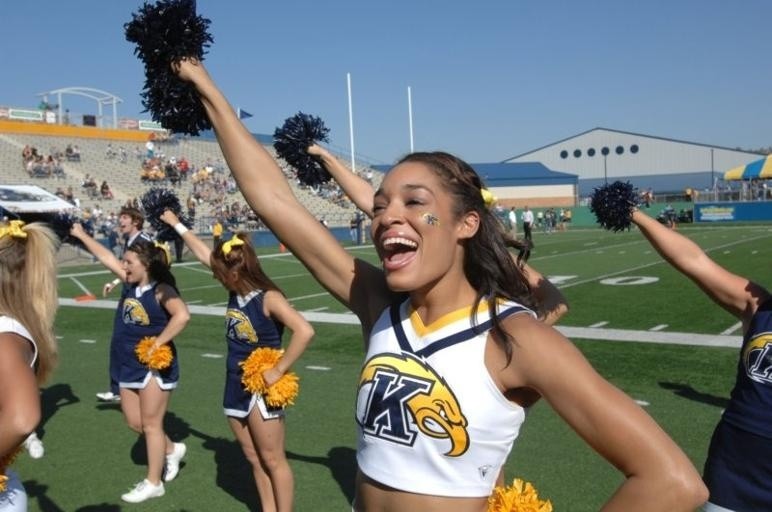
[0,136,388,240]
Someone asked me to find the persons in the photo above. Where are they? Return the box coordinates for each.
[158,206,315,512]
[68,222,186,503]
[96,209,154,401]
[508,205,571,241]
[306,142,568,325]
[0,217,58,512]
[629,204,771,512]
[170,53,710,512]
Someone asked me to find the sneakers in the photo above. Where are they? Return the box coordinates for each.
[121,477,166,505]
[95,390,121,402]
[160,441,188,482]
[23,432,46,459]
